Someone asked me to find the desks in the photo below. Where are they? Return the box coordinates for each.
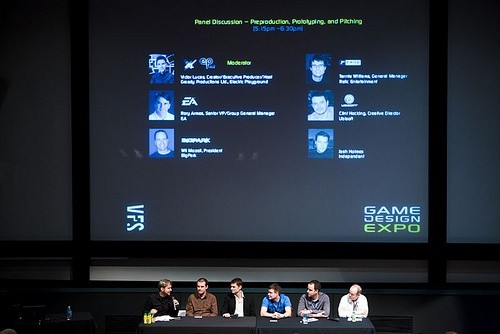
[136,316,256,334]
[257,318,375,334]
[44,312,98,334]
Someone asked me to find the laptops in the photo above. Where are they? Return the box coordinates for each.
[22,306,45,321]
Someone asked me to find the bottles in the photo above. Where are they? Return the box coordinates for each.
[144,313,148,324]
[303,314,308,325]
[67,306,72,320]
[351,312,356,323]
[147,313,152,325]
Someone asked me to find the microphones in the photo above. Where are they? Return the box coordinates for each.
[172,296,176,310]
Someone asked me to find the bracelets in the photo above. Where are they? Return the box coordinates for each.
[309,310,312,313]
[283,314,286,319]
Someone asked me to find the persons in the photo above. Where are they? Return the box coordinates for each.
[306,55,327,84]
[148,92,174,120]
[148,279,180,317]
[186,278,218,317]
[297,280,330,318]
[260,283,292,319]
[150,56,175,84]
[150,130,174,159]
[221,278,256,318]
[308,131,333,157]
[338,285,368,318]
[308,92,334,121]
